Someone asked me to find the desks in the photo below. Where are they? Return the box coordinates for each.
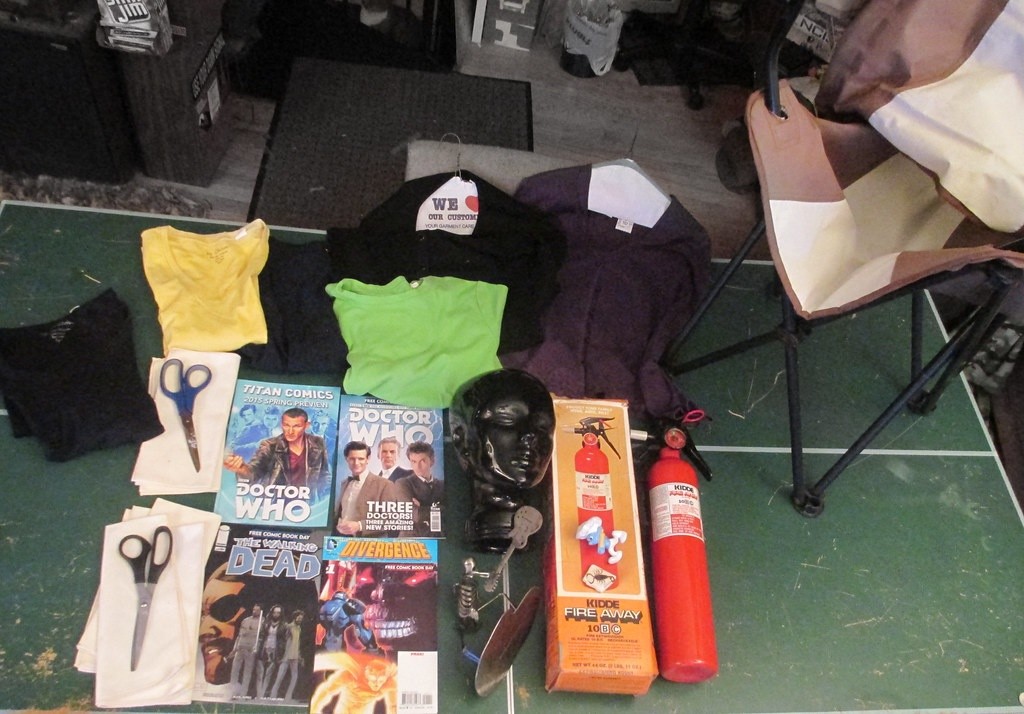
[0,202,1024,713]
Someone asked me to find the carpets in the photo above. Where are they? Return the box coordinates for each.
[246,53,535,230]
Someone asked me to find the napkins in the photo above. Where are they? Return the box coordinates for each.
[131,345,241,496]
[74,497,223,709]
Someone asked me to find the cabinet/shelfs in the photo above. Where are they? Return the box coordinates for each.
[0,0,139,184]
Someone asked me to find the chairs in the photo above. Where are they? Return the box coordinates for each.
[656,0,1024,516]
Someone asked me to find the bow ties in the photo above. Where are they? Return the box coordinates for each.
[347,474,360,483]
[424,480,434,489]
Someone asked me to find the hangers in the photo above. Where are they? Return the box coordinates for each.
[415,132,479,236]
[588,119,673,230]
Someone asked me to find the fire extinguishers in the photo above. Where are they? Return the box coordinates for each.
[562,416,621,592]
[630,407,722,684]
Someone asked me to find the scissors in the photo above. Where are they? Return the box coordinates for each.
[117,525,174,672]
[159,357,213,473]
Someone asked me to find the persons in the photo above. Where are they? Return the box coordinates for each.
[331,441,394,536]
[394,441,446,537]
[228,603,305,704]
[449,367,556,555]
[377,436,414,483]
[575,516,627,565]
[223,404,331,511]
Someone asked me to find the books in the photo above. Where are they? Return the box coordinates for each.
[334,395,447,539]
[309,536,438,714]
[191,526,333,709]
[214,379,341,527]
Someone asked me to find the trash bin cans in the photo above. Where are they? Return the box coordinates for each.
[559,0,622,78]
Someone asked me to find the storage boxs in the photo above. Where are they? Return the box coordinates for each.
[484,0,542,52]
[545,391,659,700]
